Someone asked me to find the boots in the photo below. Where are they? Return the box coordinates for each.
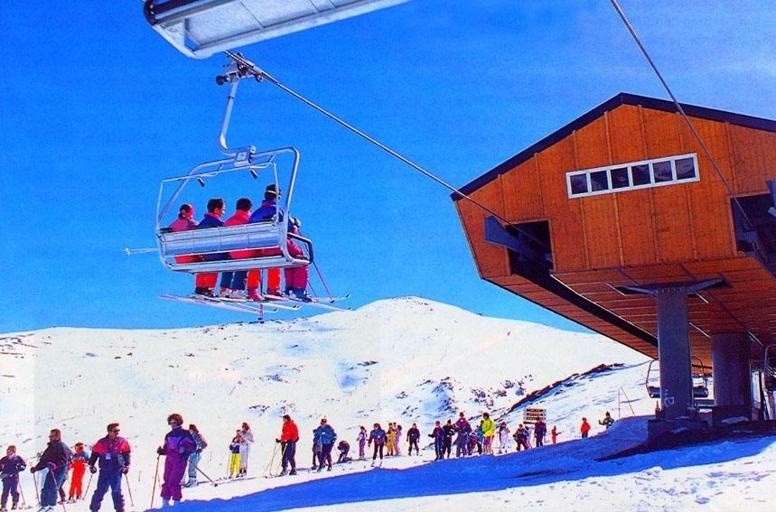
[186,474,198,488]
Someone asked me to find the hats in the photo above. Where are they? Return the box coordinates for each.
[264,183,284,199]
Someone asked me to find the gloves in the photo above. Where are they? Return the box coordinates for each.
[89,466,97,474]
[48,462,57,471]
[31,467,37,474]
[120,466,128,473]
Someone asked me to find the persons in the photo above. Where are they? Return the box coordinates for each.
[222,198,283,301]
[580,418,591,439]
[170,204,219,297]
[599,411,614,430]
[197,199,248,299]
[248,185,312,303]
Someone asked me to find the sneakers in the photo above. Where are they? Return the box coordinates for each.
[36,507,55,512]
[194,287,310,304]
